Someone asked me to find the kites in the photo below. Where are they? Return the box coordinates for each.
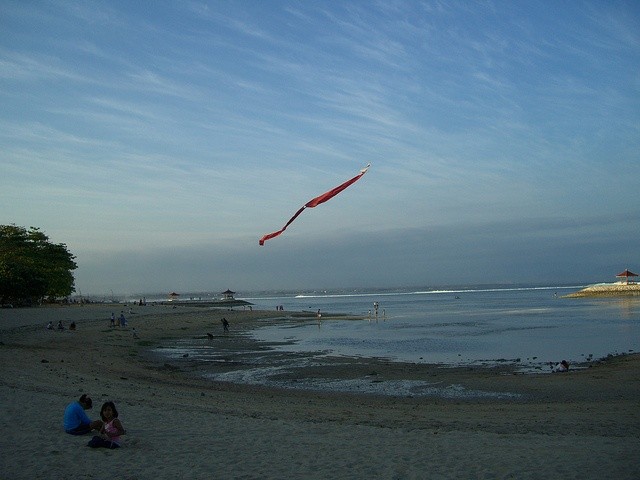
[259,162,373,246]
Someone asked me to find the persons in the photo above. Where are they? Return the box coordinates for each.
[63,393,102,434]
[70,321,75,328]
[222,318,228,332]
[128,307,136,315]
[207,333,214,339]
[58,321,64,331]
[132,329,138,338]
[47,322,53,330]
[119,314,124,326]
[110,313,116,326]
[556,359,570,372]
[87,401,124,449]
